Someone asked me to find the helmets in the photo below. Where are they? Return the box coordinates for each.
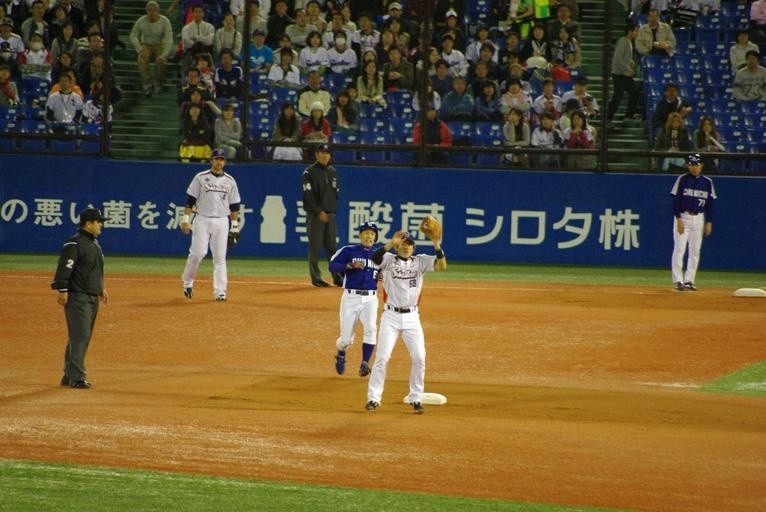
[687,154,702,166]
[361,222,376,233]
[211,149,226,158]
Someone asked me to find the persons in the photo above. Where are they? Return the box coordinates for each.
[299,31,332,77]
[734,50,766,100]
[533,80,562,116]
[502,108,530,147]
[545,5,582,36]
[284,8,319,48]
[351,13,381,64]
[501,64,531,94]
[329,223,384,377]
[177,68,212,102]
[670,154,717,292]
[750,0,766,33]
[181,149,241,303]
[365,229,447,413]
[499,0,534,41]
[386,16,411,50]
[560,98,595,131]
[475,81,502,122]
[346,85,359,115]
[441,77,475,122]
[693,117,723,170]
[51,208,108,389]
[435,15,461,42]
[498,33,526,69]
[358,60,383,105]
[654,83,692,127]
[237,0,269,41]
[328,31,358,73]
[322,13,351,50]
[440,34,467,77]
[296,70,333,119]
[551,24,582,71]
[301,145,345,288]
[215,49,244,97]
[413,102,452,147]
[337,4,357,32]
[180,41,213,86]
[0,0,125,138]
[635,9,677,57]
[181,4,214,52]
[465,24,498,60]
[383,46,413,92]
[129,2,172,95]
[181,89,211,115]
[214,13,243,57]
[468,43,499,79]
[180,105,212,159]
[730,28,760,73]
[194,55,216,98]
[501,53,519,76]
[239,29,274,72]
[605,21,640,129]
[499,76,533,112]
[375,28,406,63]
[654,111,693,173]
[272,104,302,143]
[382,2,418,46]
[268,47,301,85]
[263,0,296,50]
[273,34,299,66]
[471,62,498,84]
[562,76,600,113]
[357,47,377,75]
[521,22,548,73]
[435,0,465,28]
[562,112,595,148]
[302,101,331,144]
[412,80,441,115]
[444,29,466,55]
[531,112,564,149]
[306,0,327,34]
[327,92,358,132]
[425,46,457,80]
[214,105,242,160]
[430,59,455,102]
[229,0,272,16]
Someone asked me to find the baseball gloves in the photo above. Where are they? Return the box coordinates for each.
[420,217,442,241]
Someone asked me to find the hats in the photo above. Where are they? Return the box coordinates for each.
[442,32,456,39]
[390,3,404,10]
[311,101,323,112]
[401,232,414,242]
[318,144,330,153]
[0,15,13,27]
[362,46,375,57]
[566,98,579,108]
[446,9,457,18]
[574,74,586,83]
[80,208,111,220]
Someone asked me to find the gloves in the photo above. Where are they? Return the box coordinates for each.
[421,215,440,241]
[230,232,238,243]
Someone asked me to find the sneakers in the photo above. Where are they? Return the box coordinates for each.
[335,279,344,286]
[72,381,90,389]
[604,125,617,130]
[674,281,683,289]
[216,294,226,300]
[335,356,345,374]
[685,282,696,289]
[60,377,69,384]
[312,279,328,287]
[184,286,193,298]
[358,364,370,376]
[366,400,378,411]
[625,117,640,121]
[411,396,424,413]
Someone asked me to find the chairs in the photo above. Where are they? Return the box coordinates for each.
[391,134,415,164]
[273,87,300,104]
[359,102,385,120]
[229,101,242,117]
[447,121,473,138]
[475,134,503,167]
[19,119,49,147]
[331,131,357,163]
[359,133,388,164]
[386,91,413,107]
[638,0,766,174]
[359,117,386,135]
[22,91,46,111]
[1,103,21,121]
[52,123,76,150]
[249,115,274,132]
[251,84,272,96]
[251,129,271,160]
[251,70,268,89]
[390,104,415,120]
[249,98,273,116]
[82,122,110,154]
[24,103,44,118]
[474,122,501,140]
[1,120,16,148]
[554,80,577,95]
[530,80,551,96]
[386,117,413,137]
[443,135,473,167]
[301,70,325,87]
[23,76,50,95]
[328,73,348,91]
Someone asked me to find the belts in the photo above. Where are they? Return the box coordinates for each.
[684,210,702,214]
[384,304,417,314]
[344,289,376,296]
